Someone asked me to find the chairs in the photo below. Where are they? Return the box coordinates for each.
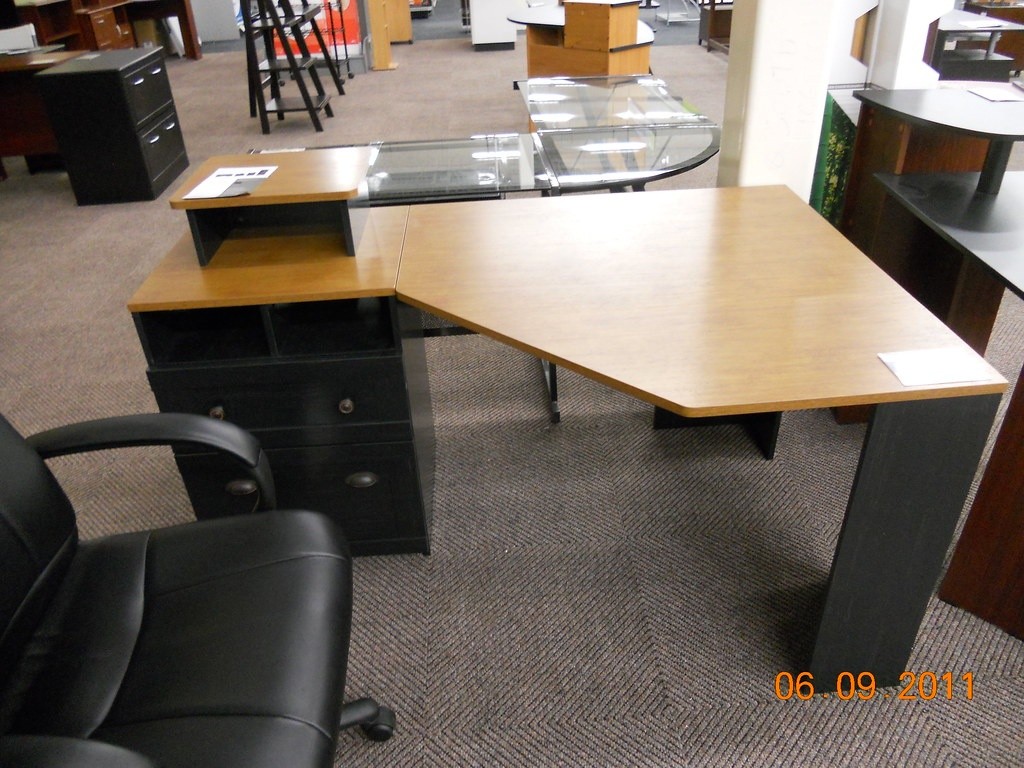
[0,414,396,768]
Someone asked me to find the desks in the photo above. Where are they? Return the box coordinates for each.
[13,0,127,50]
[922,10,1024,81]
[126,145,1007,693]
[829,81,1022,639]
[246,72,723,425]
[506,0,657,134]
[74,0,202,61]
[0,51,88,183]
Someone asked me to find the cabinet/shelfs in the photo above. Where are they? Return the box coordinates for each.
[468,0,515,52]
[368,0,398,71]
[409,0,436,19]
[698,0,733,55]
[459,0,469,35]
[131,295,437,555]
[385,0,414,44]
[33,45,189,207]
[639,0,660,9]
[240,0,355,135]
